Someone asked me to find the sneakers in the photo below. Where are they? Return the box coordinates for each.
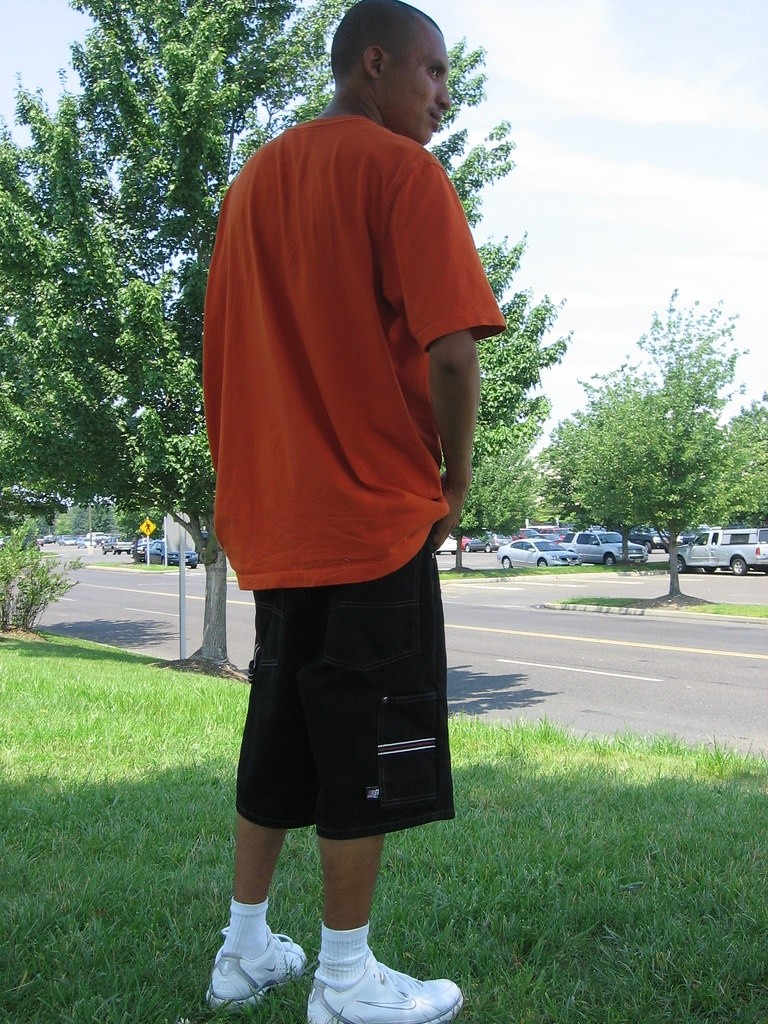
[206,922,463,1024]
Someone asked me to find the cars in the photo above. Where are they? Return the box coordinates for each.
[42,514,216,568]
[434,514,711,581]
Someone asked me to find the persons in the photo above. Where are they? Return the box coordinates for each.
[201,0,512,1021]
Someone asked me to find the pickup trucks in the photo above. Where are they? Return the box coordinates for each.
[667,528,767,576]
